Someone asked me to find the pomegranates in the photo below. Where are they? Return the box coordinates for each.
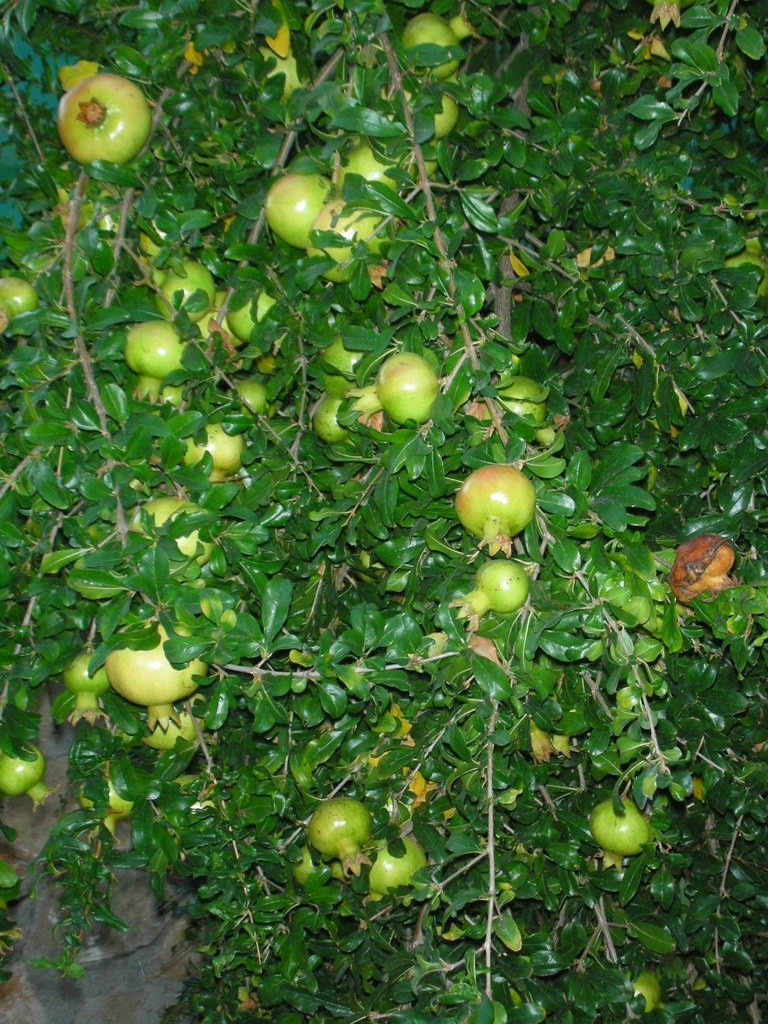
[1,16,732,891]
